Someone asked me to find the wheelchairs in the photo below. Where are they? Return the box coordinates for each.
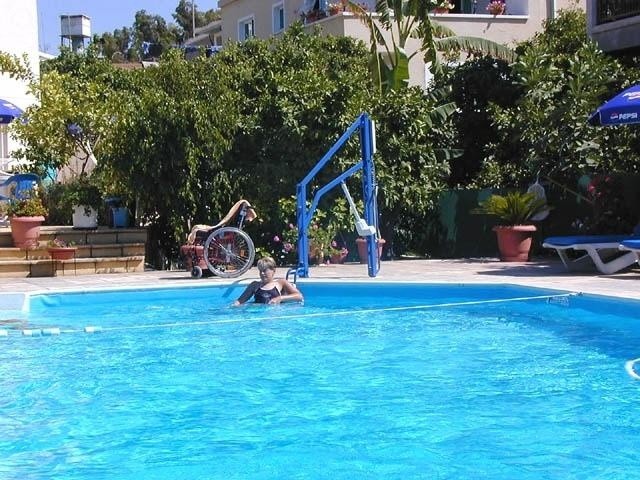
[180,200,258,279]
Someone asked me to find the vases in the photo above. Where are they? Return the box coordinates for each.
[353,238,385,263]
[48,248,78,260]
[182,244,217,272]
[111,207,131,227]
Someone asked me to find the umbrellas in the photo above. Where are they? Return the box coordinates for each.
[0,99,30,124]
[587,83,640,125]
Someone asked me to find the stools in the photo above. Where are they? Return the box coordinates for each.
[617,241,640,269]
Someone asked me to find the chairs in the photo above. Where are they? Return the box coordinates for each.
[0,172,42,202]
[540,220,640,274]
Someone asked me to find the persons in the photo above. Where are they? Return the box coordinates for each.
[231,256,304,306]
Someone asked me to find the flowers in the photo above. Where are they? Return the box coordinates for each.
[50,236,74,247]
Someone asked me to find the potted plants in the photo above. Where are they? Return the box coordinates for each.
[477,191,552,262]
[4,185,48,253]
[67,178,104,230]
